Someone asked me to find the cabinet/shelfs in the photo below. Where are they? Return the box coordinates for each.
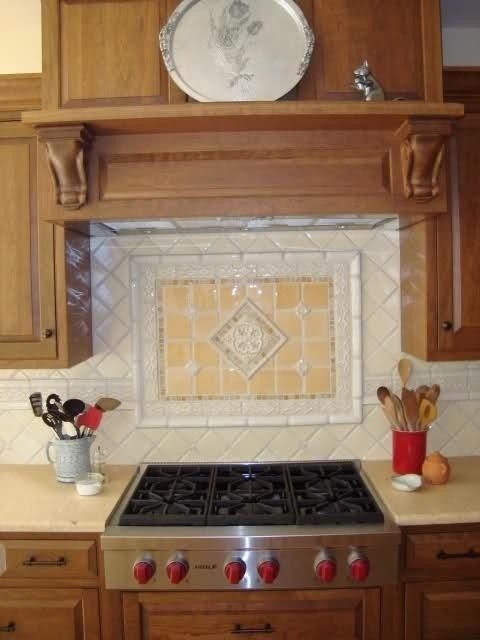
[398,66,479,361]
[1,115,99,366]
[102,588,403,639]
[0,531,118,640]
[398,520,478,638]
[39,0,442,107]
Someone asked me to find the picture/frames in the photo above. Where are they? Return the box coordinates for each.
[127,249,367,432]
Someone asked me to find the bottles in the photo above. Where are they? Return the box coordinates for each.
[92,445,105,484]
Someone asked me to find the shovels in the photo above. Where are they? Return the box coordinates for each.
[384,387,417,432]
[29,392,61,440]
[88,398,121,437]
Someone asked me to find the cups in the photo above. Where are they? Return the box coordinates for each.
[390,427,429,474]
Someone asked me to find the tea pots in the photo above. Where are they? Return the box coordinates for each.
[422,451,451,486]
[44,434,98,485]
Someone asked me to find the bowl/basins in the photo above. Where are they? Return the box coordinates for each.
[73,470,105,495]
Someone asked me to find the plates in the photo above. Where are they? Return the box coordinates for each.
[156,1,315,101]
[391,475,424,493]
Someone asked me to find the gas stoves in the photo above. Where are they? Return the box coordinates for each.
[99,458,402,593]
